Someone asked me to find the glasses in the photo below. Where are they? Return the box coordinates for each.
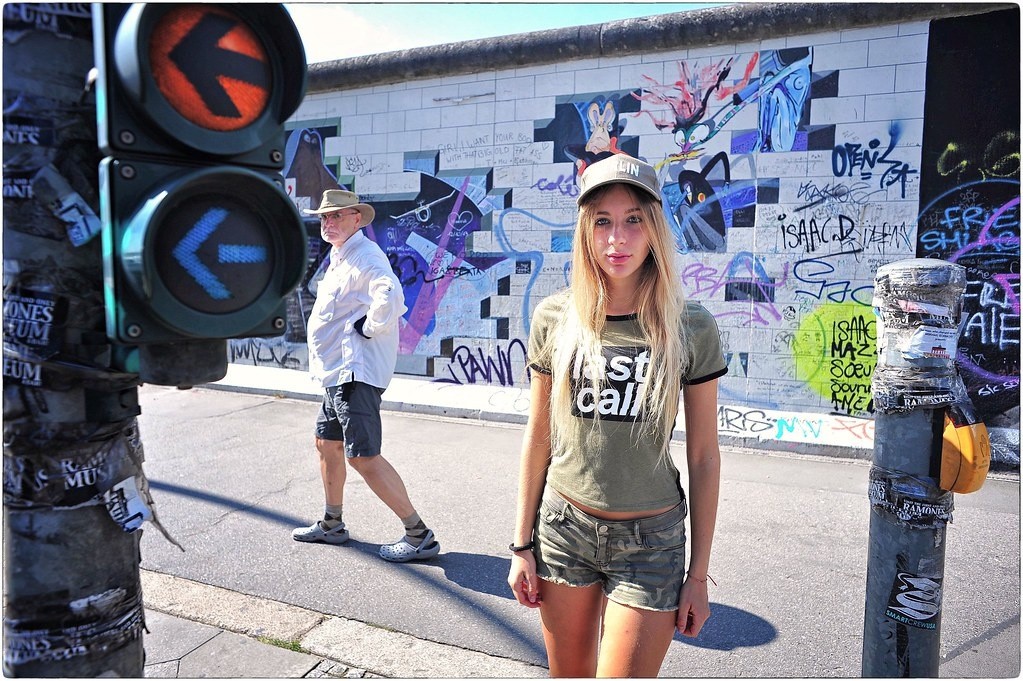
[318,212,357,222]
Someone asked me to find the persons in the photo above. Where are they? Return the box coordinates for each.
[291,190,440,563]
[508,154,729,679]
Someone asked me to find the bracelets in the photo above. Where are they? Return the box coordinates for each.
[509,542,535,552]
[686,570,717,586]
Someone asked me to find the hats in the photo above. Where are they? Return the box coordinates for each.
[302,189,376,229]
[576,152,663,212]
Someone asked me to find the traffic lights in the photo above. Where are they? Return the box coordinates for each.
[93,3,306,338]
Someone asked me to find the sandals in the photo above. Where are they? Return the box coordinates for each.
[292,520,350,544]
[379,530,441,562]
